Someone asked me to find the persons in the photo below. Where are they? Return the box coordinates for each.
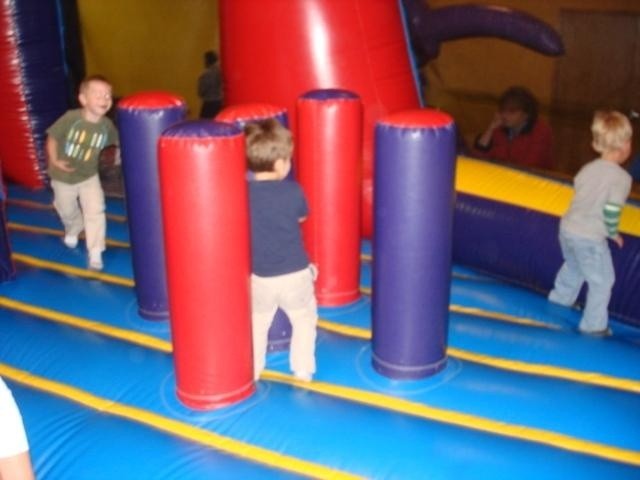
[0,377,34,480]
[43,74,122,270]
[468,85,557,172]
[547,109,634,335]
[243,118,317,382]
[197,50,222,118]
